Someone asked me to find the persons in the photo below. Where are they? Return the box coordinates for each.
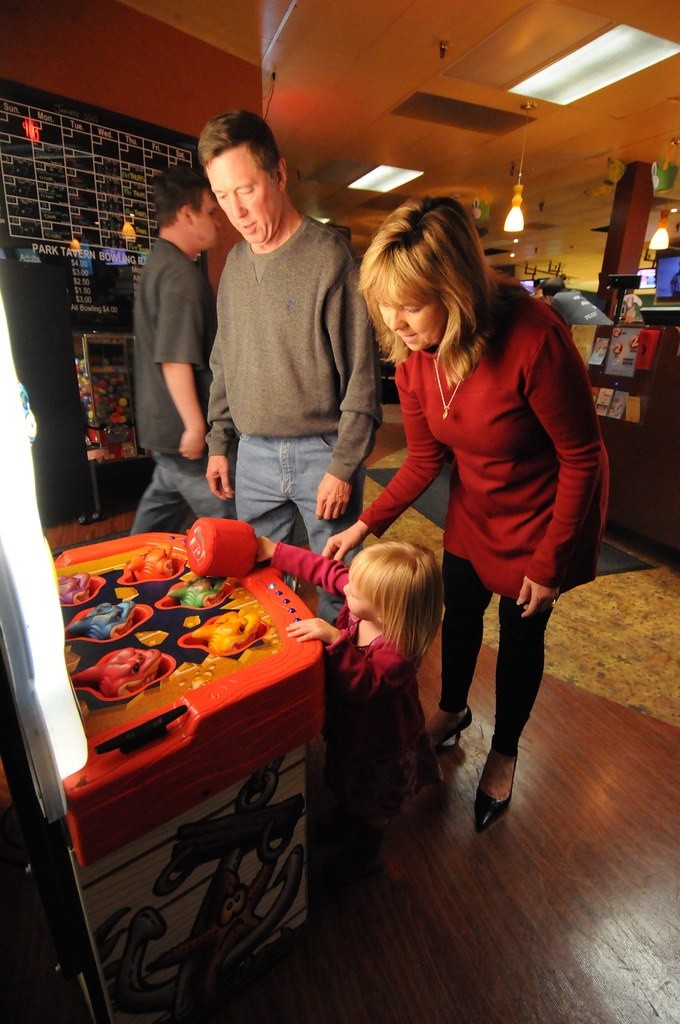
[533,276,614,324]
[321,194,608,832]
[255,536,445,890]
[130,164,237,536]
[197,111,384,625]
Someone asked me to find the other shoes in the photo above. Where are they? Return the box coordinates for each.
[316,800,354,833]
[325,847,383,887]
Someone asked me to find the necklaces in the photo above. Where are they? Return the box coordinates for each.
[433,359,462,419]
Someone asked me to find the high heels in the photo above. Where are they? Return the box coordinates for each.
[474,749,516,830]
[432,705,472,749]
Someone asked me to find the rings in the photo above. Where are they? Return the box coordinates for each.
[549,599,556,608]
